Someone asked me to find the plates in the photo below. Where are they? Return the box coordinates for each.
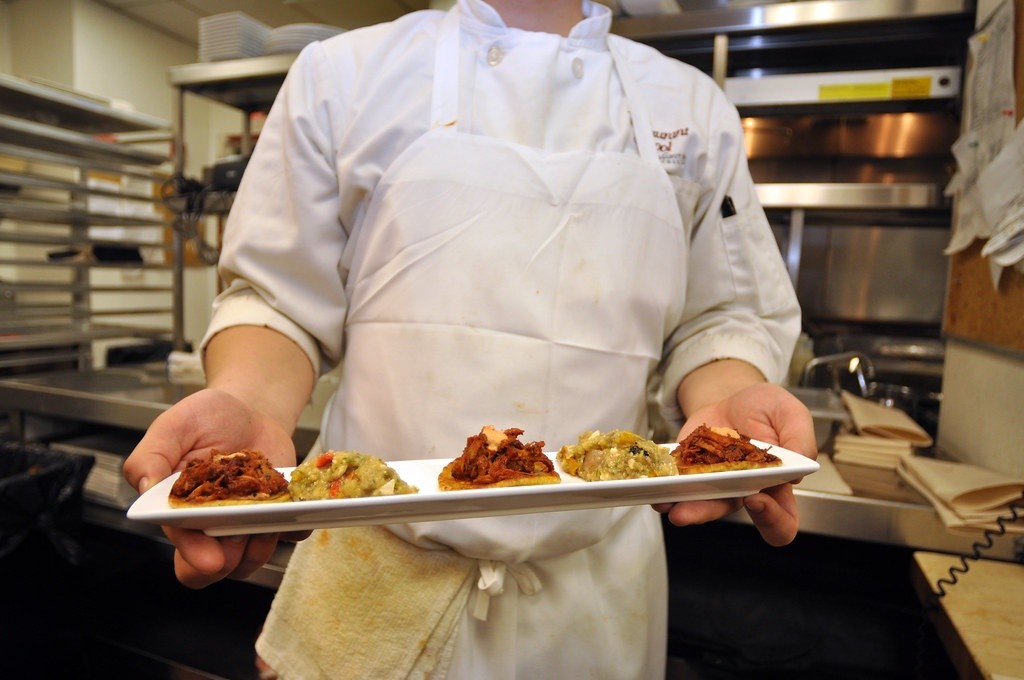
[128,438,819,536]
[198,10,348,62]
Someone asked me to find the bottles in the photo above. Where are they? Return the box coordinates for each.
[788,333,813,389]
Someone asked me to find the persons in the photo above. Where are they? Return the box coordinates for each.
[124,0,817,679]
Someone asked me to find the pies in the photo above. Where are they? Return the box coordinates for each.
[166,424,783,507]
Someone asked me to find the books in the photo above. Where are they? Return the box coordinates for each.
[834,434,913,470]
